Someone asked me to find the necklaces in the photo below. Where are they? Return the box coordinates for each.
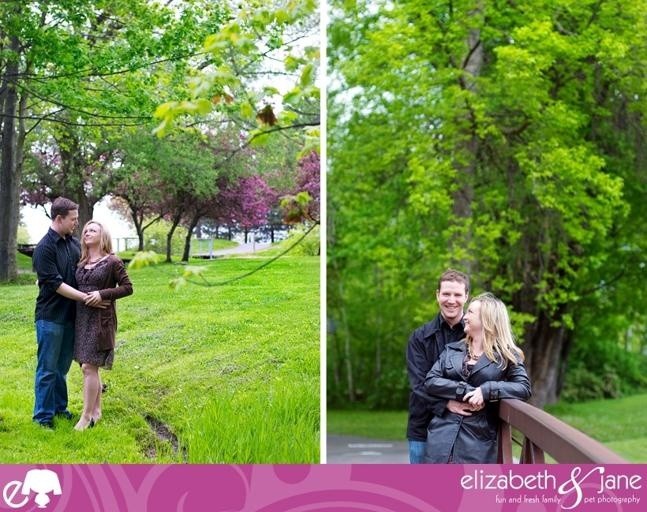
[87,258,102,265]
[469,344,481,361]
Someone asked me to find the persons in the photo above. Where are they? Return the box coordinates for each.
[31,195,112,430]
[405,268,483,464]
[35,220,134,434]
[424,291,532,464]
